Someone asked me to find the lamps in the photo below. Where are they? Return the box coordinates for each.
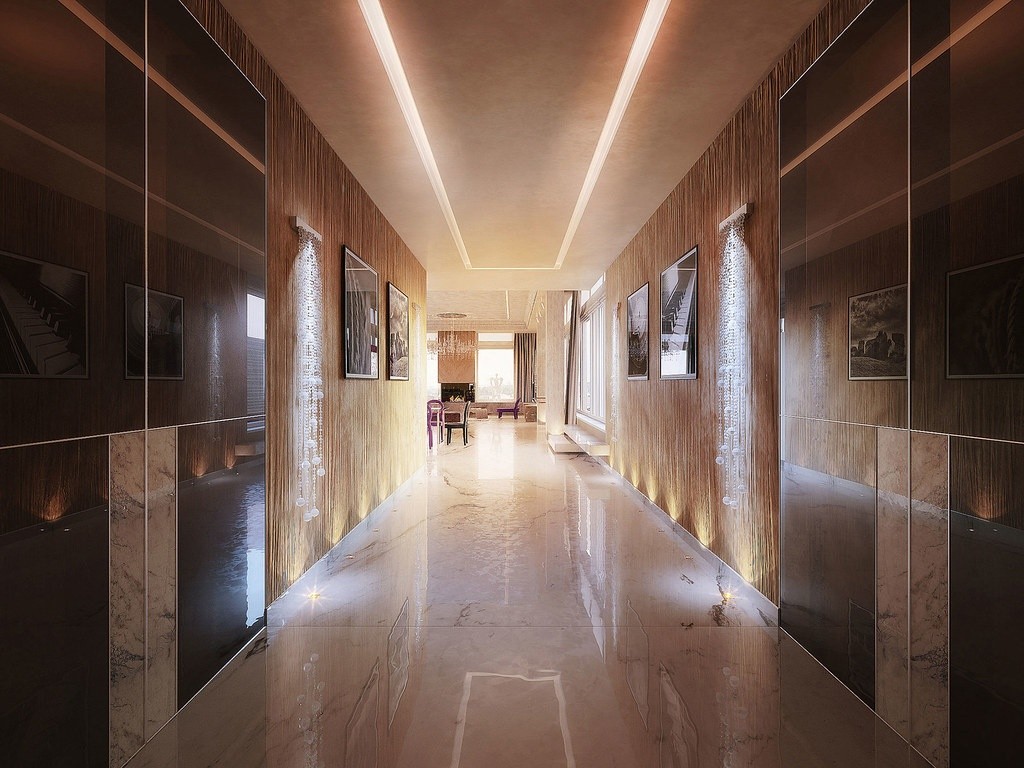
[290,215,323,248]
[427,313,479,362]
[719,202,754,234]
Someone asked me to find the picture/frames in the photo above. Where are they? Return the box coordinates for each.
[659,244,698,381]
[341,244,380,380]
[121,279,186,382]
[626,281,649,381]
[944,251,1024,380]
[0,248,92,381]
[386,280,409,381]
[845,282,914,382]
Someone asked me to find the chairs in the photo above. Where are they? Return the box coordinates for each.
[496,397,522,420]
[427,399,444,449]
[445,401,471,446]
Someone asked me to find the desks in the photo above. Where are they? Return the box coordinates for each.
[427,407,441,445]
